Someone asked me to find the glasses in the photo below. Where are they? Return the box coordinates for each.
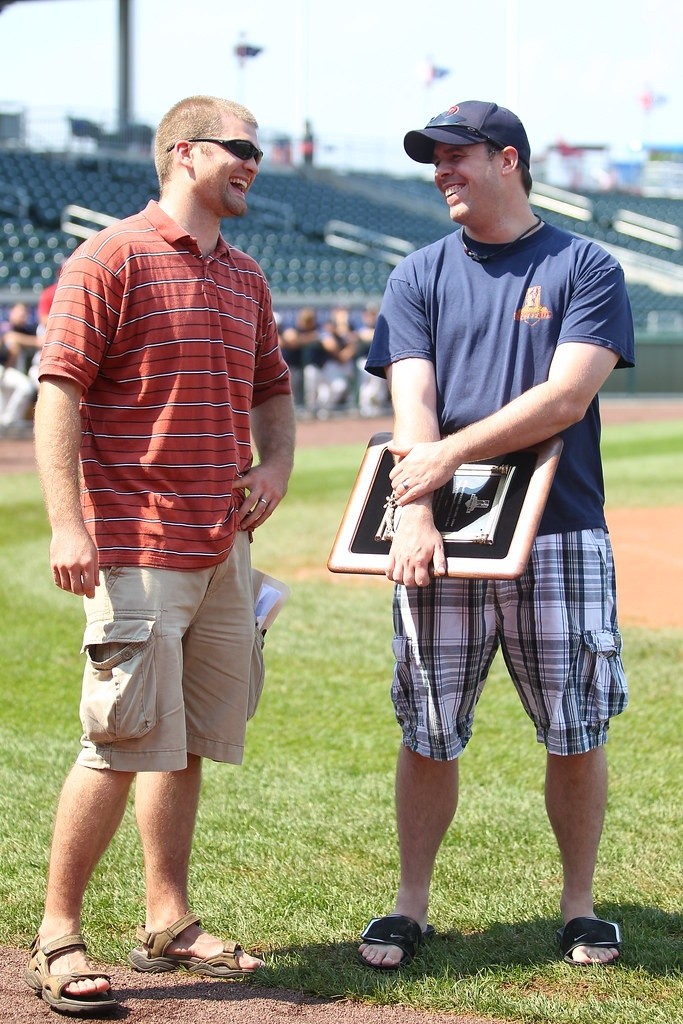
[167,138,263,164]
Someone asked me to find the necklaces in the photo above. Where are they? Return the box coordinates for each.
[459,214,542,262]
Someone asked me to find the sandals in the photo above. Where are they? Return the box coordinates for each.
[133,913,266,980]
[25,933,116,1013]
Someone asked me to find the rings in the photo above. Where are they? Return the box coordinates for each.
[402,481,411,492]
[259,498,269,505]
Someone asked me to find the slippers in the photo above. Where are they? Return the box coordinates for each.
[552,919,618,968]
[357,915,436,969]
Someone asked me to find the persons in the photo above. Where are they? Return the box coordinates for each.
[355,98,622,970]
[301,120,316,167]
[21,94,299,1012]
[1,272,399,428]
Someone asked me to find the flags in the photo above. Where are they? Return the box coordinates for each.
[643,94,667,110]
[236,44,261,56]
[430,66,448,78]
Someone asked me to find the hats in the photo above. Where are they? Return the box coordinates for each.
[404,99,531,165]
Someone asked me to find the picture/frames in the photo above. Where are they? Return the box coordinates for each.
[329,431,564,580]
